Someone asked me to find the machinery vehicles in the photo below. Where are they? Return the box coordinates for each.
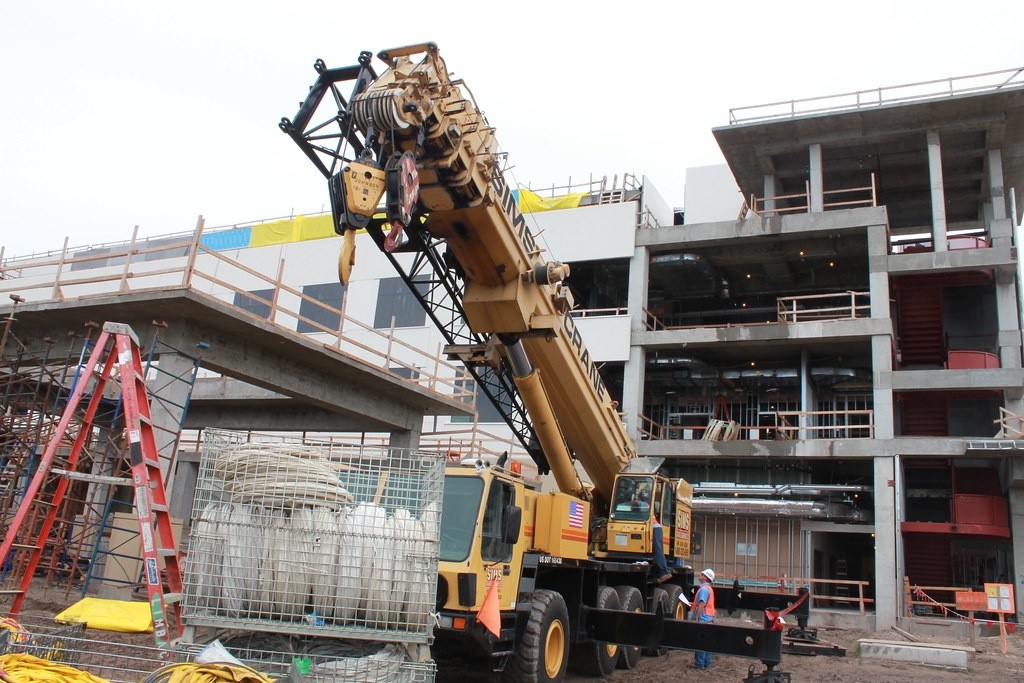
[279,42,695,683]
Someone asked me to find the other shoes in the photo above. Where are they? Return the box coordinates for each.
[705,666,711,671]
[657,574,672,583]
[690,664,705,670]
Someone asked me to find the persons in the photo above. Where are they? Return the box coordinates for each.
[690,568,715,668]
[622,490,672,584]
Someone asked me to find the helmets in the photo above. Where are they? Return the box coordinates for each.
[637,493,649,498]
[701,568,715,582]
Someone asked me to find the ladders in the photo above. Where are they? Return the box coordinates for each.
[0,321,186,672]
[737,198,749,219]
